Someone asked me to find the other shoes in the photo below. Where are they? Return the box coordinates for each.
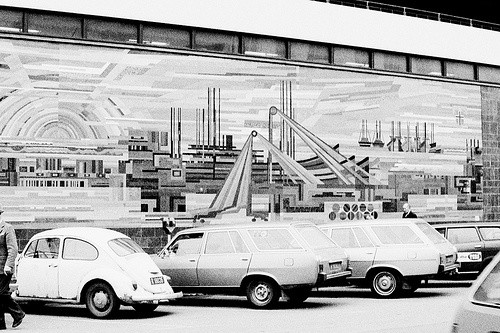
[12,312,25,328]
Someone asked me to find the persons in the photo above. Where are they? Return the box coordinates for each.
[0,210,26,330]
[402,203,417,219]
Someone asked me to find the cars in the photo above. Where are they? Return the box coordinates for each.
[451,255,499,333]
[151,223,353,308]
[315,218,461,299]
[10,226,183,318]
[429,222,500,276]
